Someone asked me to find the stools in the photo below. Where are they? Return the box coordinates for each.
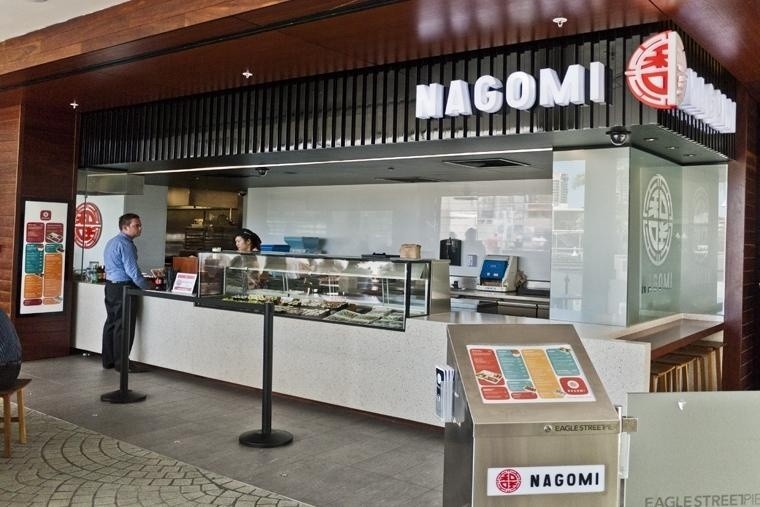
[0,378,33,459]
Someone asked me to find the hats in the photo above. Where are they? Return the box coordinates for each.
[235,229,261,246]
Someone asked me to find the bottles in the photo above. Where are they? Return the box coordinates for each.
[154,270,166,290]
[86,263,107,285]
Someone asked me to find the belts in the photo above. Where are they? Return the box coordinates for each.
[105,279,133,286]
[0,359,22,367]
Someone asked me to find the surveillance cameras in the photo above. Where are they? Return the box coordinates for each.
[254,167,271,176]
[605,130,633,147]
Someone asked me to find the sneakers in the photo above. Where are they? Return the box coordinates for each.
[128,366,145,372]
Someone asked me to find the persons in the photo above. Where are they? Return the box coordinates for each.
[234,228,268,290]
[101,212,154,372]
[0,297,23,393]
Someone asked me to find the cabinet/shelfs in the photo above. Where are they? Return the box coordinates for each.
[195,249,431,332]
